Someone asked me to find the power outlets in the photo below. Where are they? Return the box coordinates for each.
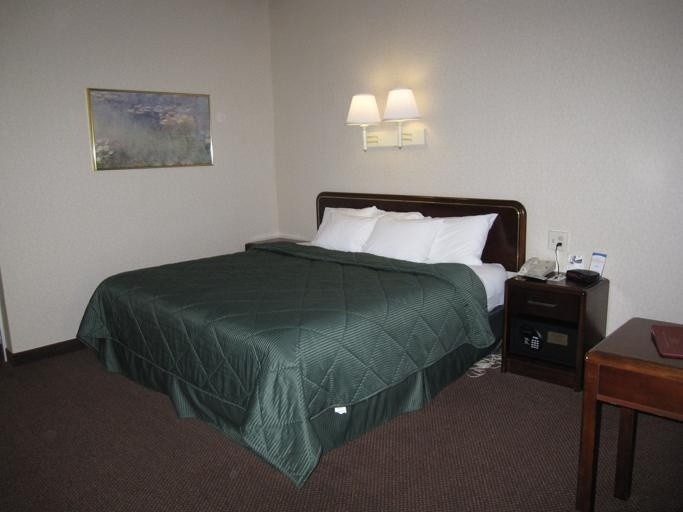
[548,230,569,251]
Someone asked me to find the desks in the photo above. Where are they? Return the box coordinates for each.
[575,316,683,512]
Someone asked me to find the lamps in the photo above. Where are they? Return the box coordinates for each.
[344,94,382,152]
[382,89,425,149]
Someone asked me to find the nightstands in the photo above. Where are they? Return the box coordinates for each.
[244,236,309,251]
[502,271,610,392]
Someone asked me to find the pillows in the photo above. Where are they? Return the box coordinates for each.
[311,206,382,253]
[361,213,499,266]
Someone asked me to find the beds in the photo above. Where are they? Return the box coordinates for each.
[82,193,527,483]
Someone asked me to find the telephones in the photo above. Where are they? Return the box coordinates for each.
[516,257,556,281]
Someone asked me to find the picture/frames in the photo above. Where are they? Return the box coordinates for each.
[85,88,213,172]
